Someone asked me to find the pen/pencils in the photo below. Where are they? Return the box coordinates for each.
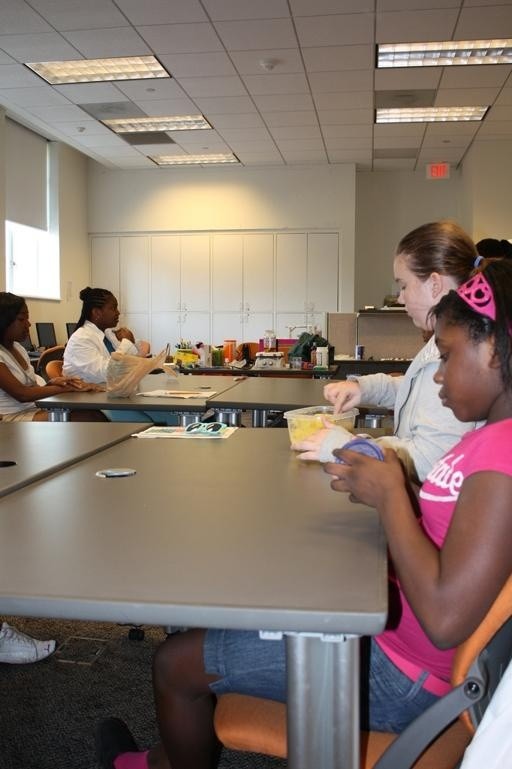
[174,337,191,349]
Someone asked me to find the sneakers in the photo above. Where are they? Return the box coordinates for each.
[97,716,140,768]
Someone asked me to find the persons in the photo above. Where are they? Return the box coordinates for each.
[290,222,487,491]
[95,255,512,769]
[0,291,111,422]
[62,286,201,426]
[475,237,512,258]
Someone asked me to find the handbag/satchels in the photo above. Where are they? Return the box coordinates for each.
[287,333,335,364]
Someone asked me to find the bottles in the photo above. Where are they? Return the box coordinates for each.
[355,344,365,360]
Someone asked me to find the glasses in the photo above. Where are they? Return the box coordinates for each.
[185,421,228,434]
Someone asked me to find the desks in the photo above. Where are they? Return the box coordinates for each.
[207,376,357,428]
[173,365,340,379]
[2,423,390,769]
[0,420,151,494]
[35,371,245,425]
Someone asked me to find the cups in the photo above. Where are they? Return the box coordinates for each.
[336,437,413,471]
[224,339,238,368]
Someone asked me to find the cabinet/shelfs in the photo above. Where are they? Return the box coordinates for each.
[88,230,149,342]
[150,232,211,355]
[276,231,340,340]
[211,231,276,349]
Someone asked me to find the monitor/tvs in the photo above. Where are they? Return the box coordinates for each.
[66,323,78,338]
[20,332,34,350]
[36,323,57,347]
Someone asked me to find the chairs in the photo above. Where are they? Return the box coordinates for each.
[37,343,67,384]
[210,572,512,768]
